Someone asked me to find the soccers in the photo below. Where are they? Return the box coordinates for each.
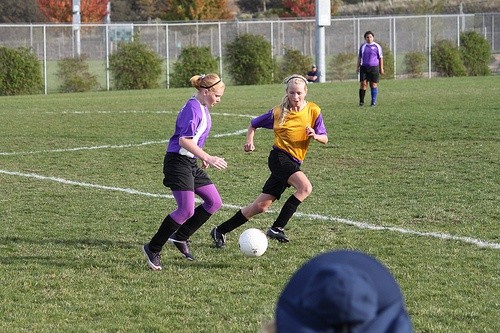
[239,228,268,258]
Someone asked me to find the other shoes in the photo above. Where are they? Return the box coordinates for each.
[359,102,364,106]
[371,102,375,107]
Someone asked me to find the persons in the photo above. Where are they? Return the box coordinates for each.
[303,64,321,82]
[210,75,328,248]
[356,30,384,107]
[259,251,415,333]
[142,73,227,271]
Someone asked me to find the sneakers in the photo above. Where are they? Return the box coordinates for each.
[142,243,162,270]
[267,227,289,243]
[210,226,226,249]
[168,233,195,261]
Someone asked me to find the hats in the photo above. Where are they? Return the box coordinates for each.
[275,250,413,333]
[312,65,316,68]
